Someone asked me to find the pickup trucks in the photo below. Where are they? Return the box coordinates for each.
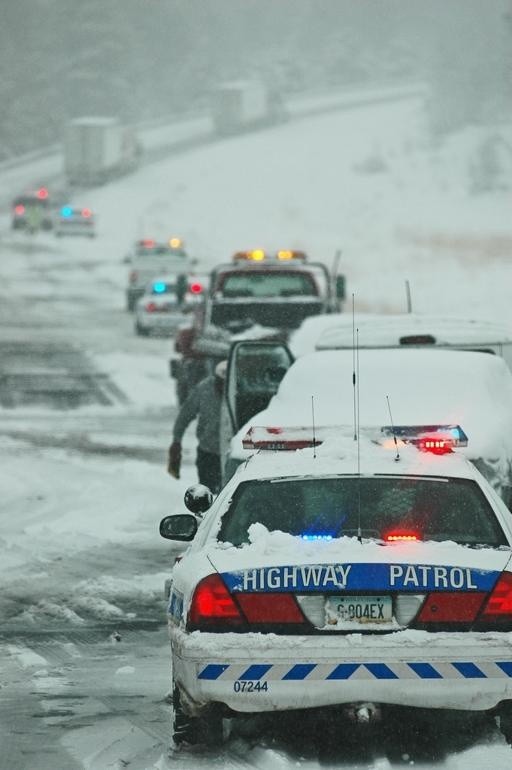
[171,248,344,413]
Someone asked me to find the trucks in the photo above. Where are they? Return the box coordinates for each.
[208,73,288,135]
[63,116,142,192]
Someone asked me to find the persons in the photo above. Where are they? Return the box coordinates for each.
[169,358,229,492]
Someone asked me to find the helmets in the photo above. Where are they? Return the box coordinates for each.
[215,359,230,379]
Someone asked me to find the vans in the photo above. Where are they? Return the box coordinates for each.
[10,189,55,232]
[222,309,512,435]
[122,237,194,311]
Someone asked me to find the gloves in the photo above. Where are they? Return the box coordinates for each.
[168,443,183,480]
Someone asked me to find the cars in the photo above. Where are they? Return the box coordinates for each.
[49,208,101,235]
[135,273,204,336]
[161,442,512,740]
[226,349,512,499]
